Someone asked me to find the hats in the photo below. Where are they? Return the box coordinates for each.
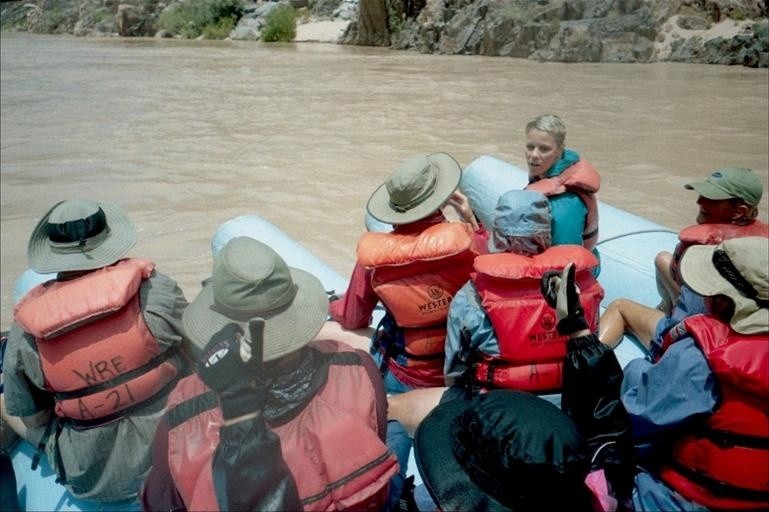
[25,196,139,276]
[679,235,769,335]
[366,149,463,225]
[492,188,554,239]
[682,165,764,207]
[413,388,597,512]
[177,235,327,363]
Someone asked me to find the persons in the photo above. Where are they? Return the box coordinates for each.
[1,113,768,512]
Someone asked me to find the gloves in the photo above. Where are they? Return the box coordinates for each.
[540,262,590,334]
[196,317,274,421]
